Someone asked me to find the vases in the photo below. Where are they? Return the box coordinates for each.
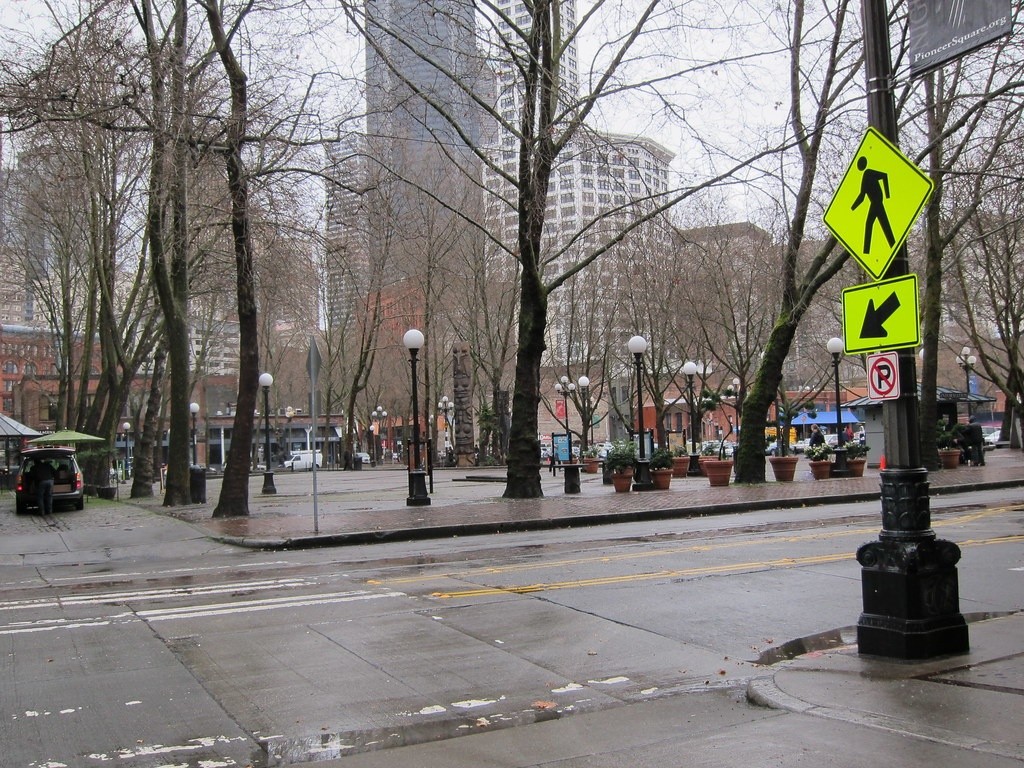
[809,460,872,481]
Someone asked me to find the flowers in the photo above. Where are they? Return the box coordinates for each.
[806,439,872,462]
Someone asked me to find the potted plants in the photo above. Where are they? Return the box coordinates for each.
[78,447,119,499]
[571,388,739,493]
[769,402,816,482]
[936,419,966,469]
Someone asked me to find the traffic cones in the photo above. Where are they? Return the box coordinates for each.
[547,458,549,464]
[879,454,885,469]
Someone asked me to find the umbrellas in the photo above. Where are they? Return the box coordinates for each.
[27,427,106,446]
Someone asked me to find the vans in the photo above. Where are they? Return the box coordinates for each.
[278,454,322,472]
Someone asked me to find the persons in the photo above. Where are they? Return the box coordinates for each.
[31,461,55,516]
[810,423,866,464]
[951,415,986,467]
[475,445,479,459]
[112,456,122,484]
[548,445,561,473]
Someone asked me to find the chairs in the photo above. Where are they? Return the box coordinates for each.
[27,463,69,480]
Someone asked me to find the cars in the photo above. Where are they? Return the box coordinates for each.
[353,453,370,464]
[765,441,778,455]
[393,453,398,459]
[686,439,738,457]
[982,427,1001,446]
[792,431,865,452]
[540,443,615,459]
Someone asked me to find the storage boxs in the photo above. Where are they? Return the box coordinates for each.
[53,484,73,493]
[59,471,69,479]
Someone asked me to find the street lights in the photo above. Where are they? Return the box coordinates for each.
[579,375,590,454]
[403,329,431,506]
[827,337,850,477]
[259,373,276,493]
[373,406,387,463]
[555,376,576,464]
[684,362,703,476]
[190,403,199,467]
[956,347,977,416]
[370,425,376,467]
[123,422,130,480]
[628,336,654,491]
[286,406,294,455]
[727,378,741,445]
[437,396,453,456]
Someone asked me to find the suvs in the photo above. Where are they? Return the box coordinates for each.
[221,461,266,473]
[15,447,85,514]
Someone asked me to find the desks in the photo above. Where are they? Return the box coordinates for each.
[552,464,588,494]
